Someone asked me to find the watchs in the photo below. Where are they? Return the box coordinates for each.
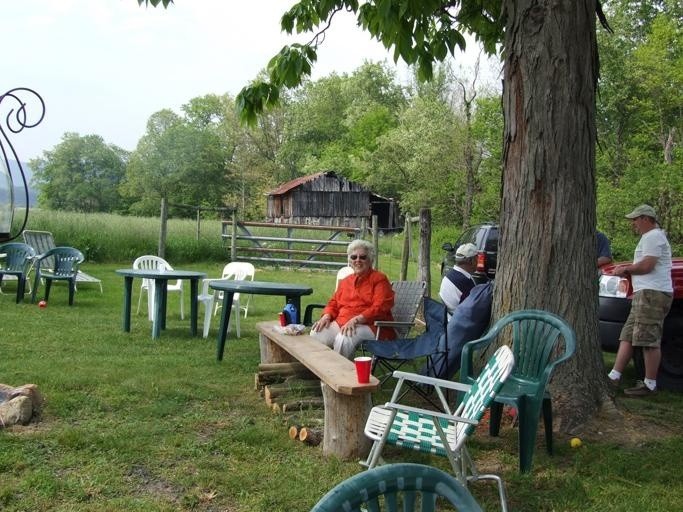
[624,266,629,275]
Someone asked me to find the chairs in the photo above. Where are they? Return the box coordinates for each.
[0,229,104,306]
[302,267,355,327]
[134,255,185,321]
[359,345,514,512]
[198,269,248,338]
[212,262,255,319]
[357,281,428,382]
[308,462,488,512]
[361,296,451,416]
[456,309,577,475]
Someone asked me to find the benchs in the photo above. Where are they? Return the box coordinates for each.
[257,320,380,462]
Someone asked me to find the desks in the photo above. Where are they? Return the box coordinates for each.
[117,269,206,338]
[208,280,313,361]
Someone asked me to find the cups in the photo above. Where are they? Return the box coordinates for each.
[354,356,372,383]
[278,313,286,327]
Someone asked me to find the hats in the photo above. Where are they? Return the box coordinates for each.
[625,204,656,219]
[456,243,483,258]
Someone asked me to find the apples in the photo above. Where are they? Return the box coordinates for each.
[39,301,47,307]
[572,438,581,448]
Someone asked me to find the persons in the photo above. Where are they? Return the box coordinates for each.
[437,242,483,322]
[595,228,612,267]
[606,204,674,396]
[309,239,396,361]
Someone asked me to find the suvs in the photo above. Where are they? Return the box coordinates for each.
[437,222,500,280]
[595,246,683,394]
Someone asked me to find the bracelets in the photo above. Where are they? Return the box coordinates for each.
[354,317,359,324]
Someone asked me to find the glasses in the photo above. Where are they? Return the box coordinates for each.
[351,255,367,259]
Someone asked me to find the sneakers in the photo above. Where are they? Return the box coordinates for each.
[607,376,620,387]
[624,380,658,396]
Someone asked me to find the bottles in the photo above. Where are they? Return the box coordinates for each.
[283,298,299,324]
